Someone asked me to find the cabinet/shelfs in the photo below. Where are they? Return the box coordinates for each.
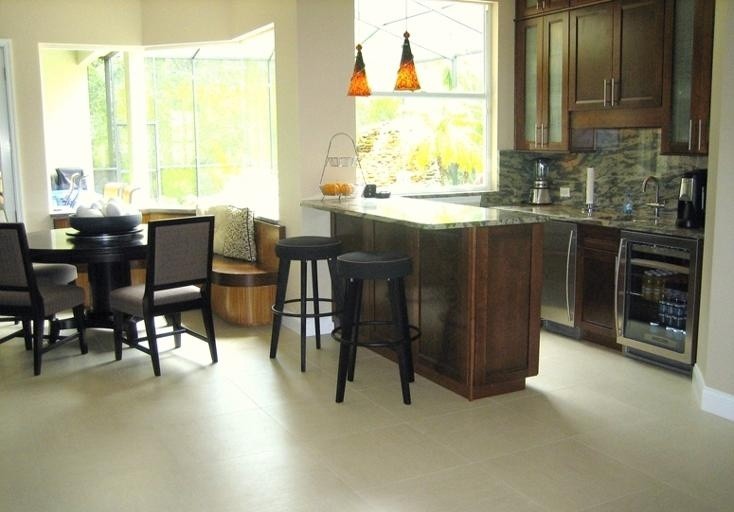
[511,13,598,156]
[576,222,621,349]
[568,0,671,128]
[656,0,716,157]
[511,2,570,18]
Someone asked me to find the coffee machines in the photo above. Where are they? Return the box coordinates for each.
[675,169,706,227]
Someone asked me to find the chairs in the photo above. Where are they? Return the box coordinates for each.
[110,215,219,378]
[0,223,87,375]
[1,208,80,348]
[50,167,142,216]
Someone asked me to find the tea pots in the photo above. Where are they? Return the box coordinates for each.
[75,198,135,217]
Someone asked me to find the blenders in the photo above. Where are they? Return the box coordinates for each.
[527,158,554,205]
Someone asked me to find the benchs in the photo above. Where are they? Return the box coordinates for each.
[49,207,289,327]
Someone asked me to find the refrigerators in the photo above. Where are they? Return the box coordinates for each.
[612,229,703,376]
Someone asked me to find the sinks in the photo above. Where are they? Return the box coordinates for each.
[597,214,650,222]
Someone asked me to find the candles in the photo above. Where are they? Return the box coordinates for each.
[585,166,595,205]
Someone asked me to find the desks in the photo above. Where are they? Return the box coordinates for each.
[298,195,551,403]
[22,223,177,330]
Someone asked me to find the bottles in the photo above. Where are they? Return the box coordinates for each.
[623,192,633,215]
[639,266,683,303]
[654,296,689,329]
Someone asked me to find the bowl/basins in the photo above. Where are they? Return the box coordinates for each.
[68,213,145,233]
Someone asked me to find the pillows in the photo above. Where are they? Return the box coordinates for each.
[204,206,257,262]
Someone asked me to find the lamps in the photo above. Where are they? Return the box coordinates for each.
[348,0,371,96]
[394,1,422,93]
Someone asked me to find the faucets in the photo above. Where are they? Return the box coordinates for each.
[641,174,664,216]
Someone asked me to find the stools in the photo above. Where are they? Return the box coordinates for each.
[330,251,423,406]
[268,237,348,372]
